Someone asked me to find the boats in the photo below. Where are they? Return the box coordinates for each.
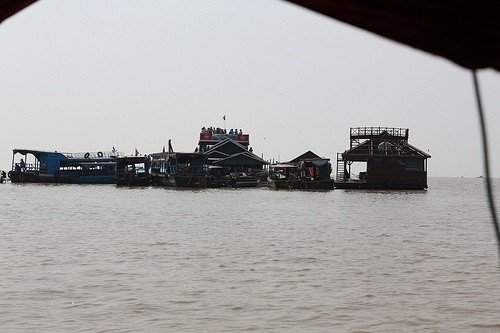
[7,133,335,192]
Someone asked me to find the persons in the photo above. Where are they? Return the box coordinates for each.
[202,127,242,134]
[0,158,25,185]
